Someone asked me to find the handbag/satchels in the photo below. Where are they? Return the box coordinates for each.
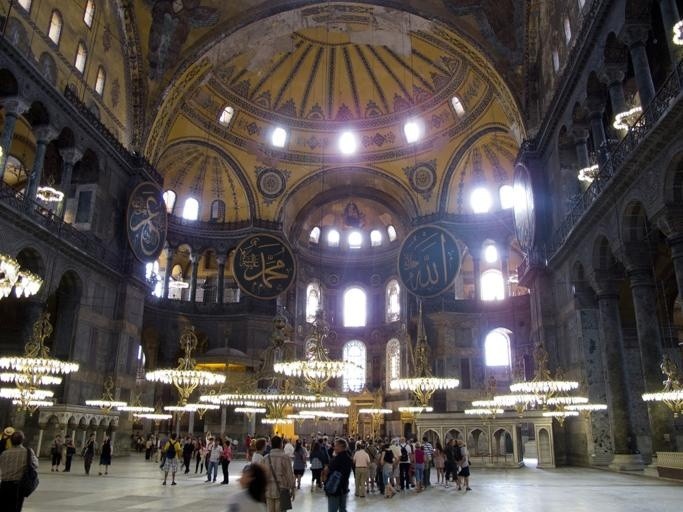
[280,489,292,510]
[81,447,88,456]
[326,471,342,494]
[22,465,39,497]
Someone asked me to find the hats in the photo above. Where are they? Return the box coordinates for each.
[4,427,15,436]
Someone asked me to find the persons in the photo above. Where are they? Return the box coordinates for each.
[310,435,472,511]
[229,434,308,511]
[0,427,39,512]
[50,435,74,472]
[136,431,232,485]
[83,433,111,475]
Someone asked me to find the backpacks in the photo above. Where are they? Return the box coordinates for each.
[0,434,10,454]
[167,441,177,459]
[400,444,408,462]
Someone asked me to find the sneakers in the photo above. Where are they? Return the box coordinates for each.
[163,482,166,484]
[172,483,175,484]
[467,488,471,489]
[206,480,228,483]
